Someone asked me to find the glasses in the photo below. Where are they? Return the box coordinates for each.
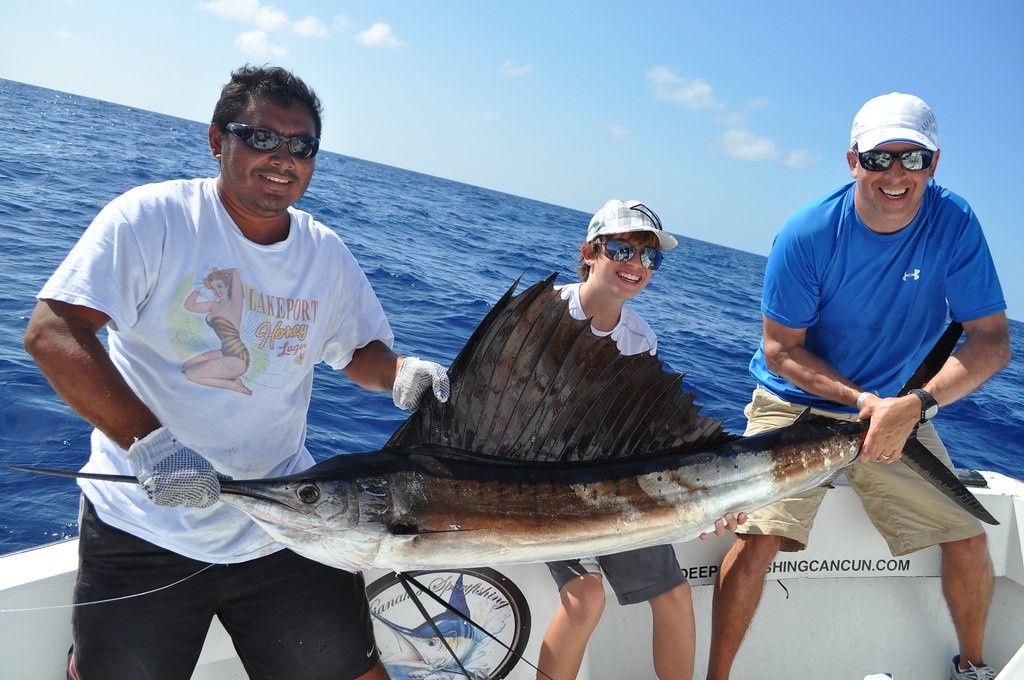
[853,147,935,172]
[225,122,321,159]
[594,239,664,270]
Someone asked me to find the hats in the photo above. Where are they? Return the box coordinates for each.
[587,200,678,251]
[850,91,937,153]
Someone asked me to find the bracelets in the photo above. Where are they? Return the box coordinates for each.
[857,390,880,411]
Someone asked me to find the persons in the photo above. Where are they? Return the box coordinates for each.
[706,92,1011,680]
[536,199,746,680]
[24,65,450,680]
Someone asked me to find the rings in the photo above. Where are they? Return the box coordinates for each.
[881,454,890,460]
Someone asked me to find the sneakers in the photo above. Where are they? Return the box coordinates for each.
[951,655,996,680]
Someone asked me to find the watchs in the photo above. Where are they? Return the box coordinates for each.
[908,388,939,424]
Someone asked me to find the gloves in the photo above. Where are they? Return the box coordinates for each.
[392,356,450,413]
[124,427,233,509]
